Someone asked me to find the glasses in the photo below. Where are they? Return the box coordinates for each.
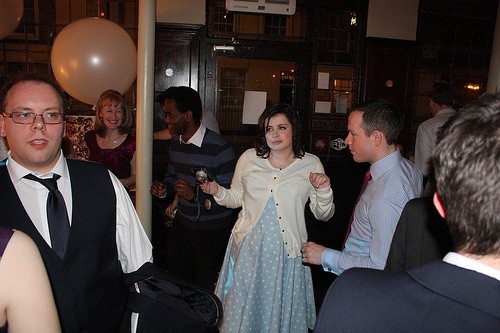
[162,107,184,119]
[1,112,66,124]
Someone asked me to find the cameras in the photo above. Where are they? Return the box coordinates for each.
[190,165,214,184]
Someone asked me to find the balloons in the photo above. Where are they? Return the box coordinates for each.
[0,0,24,40]
[51,17,137,107]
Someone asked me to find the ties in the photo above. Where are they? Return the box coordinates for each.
[342,170,371,251]
[23,173,70,260]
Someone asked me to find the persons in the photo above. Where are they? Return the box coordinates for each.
[310,91,500,333]
[415,84,460,188]
[200,102,336,333]
[0,226,64,333]
[84,88,137,192]
[0,71,222,333]
[301,99,425,276]
[150,86,238,292]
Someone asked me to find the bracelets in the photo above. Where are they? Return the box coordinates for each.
[124,179,129,188]
